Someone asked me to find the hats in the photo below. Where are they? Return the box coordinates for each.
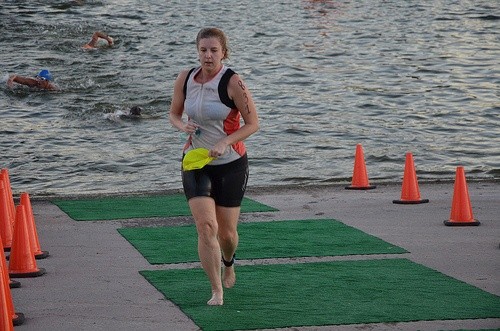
[130,106,144,116]
[37,70,53,81]
[99,39,108,46]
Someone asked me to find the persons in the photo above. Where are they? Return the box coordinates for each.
[8,69,58,92]
[129,106,144,119]
[83,31,113,48]
[170,27,259,306]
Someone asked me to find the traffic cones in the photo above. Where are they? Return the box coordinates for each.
[393,152,429,204]
[345,143,376,190]
[0,168,49,331]
[443,167,480,226]
